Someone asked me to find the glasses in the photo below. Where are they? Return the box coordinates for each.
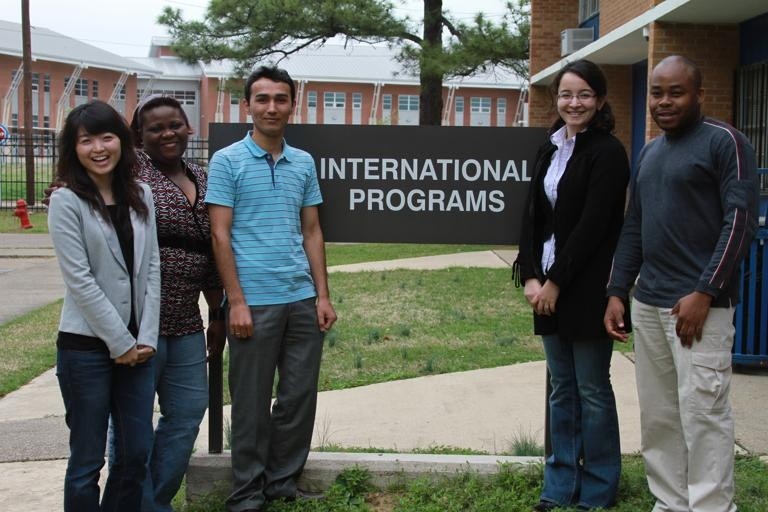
[555,90,597,102]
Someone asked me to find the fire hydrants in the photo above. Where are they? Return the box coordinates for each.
[13,197,34,230]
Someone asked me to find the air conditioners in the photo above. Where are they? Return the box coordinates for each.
[560,27,594,57]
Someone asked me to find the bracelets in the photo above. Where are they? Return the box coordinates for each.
[207,307,225,323]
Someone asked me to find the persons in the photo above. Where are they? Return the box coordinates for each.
[516,57,631,512]
[44,99,162,512]
[203,67,337,512]
[600,52,761,512]
[128,92,228,511]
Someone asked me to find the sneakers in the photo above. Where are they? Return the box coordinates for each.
[533,499,558,512]
